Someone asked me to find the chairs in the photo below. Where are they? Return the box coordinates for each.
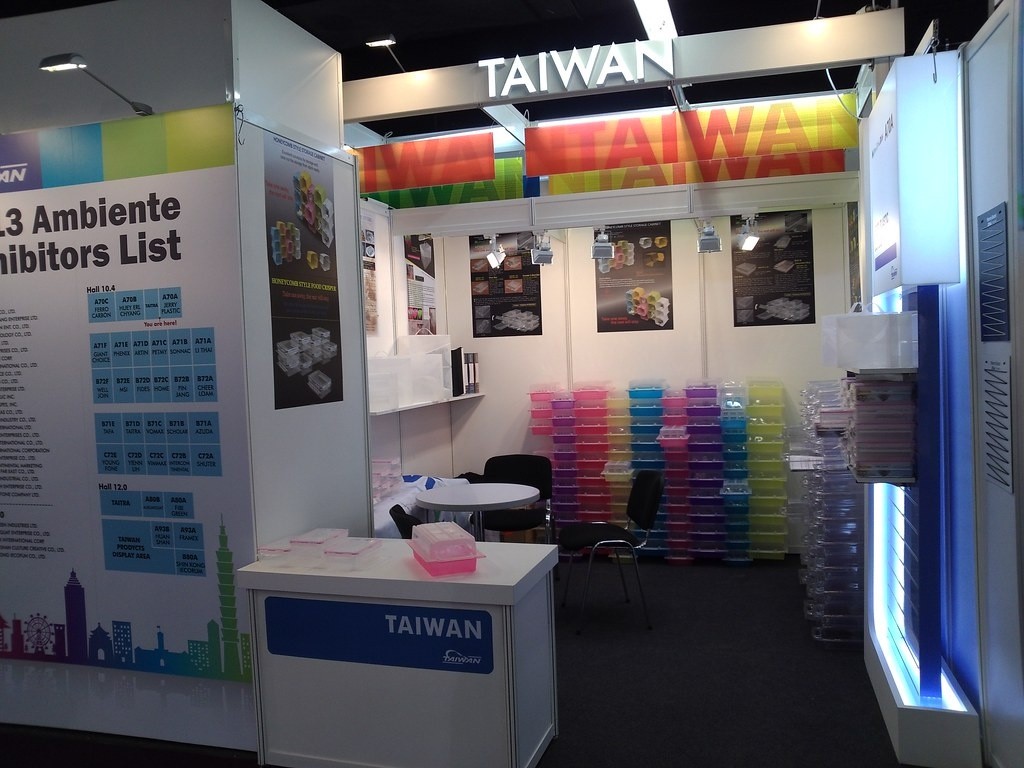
[557,468,665,634]
[388,504,421,540]
[469,454,556,545]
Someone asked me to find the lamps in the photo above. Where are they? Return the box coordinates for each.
[39,53,152,118]
[483,215,759,268]
[365,32,408,73]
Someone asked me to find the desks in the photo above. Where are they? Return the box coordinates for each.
[416,483,540,541]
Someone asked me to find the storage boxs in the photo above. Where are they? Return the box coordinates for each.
[257,522,485,577]
[784,372,867,647]
[277,327,338,396]
[529,378,789,564]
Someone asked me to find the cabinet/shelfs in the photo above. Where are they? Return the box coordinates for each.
[234,536,560,767]
